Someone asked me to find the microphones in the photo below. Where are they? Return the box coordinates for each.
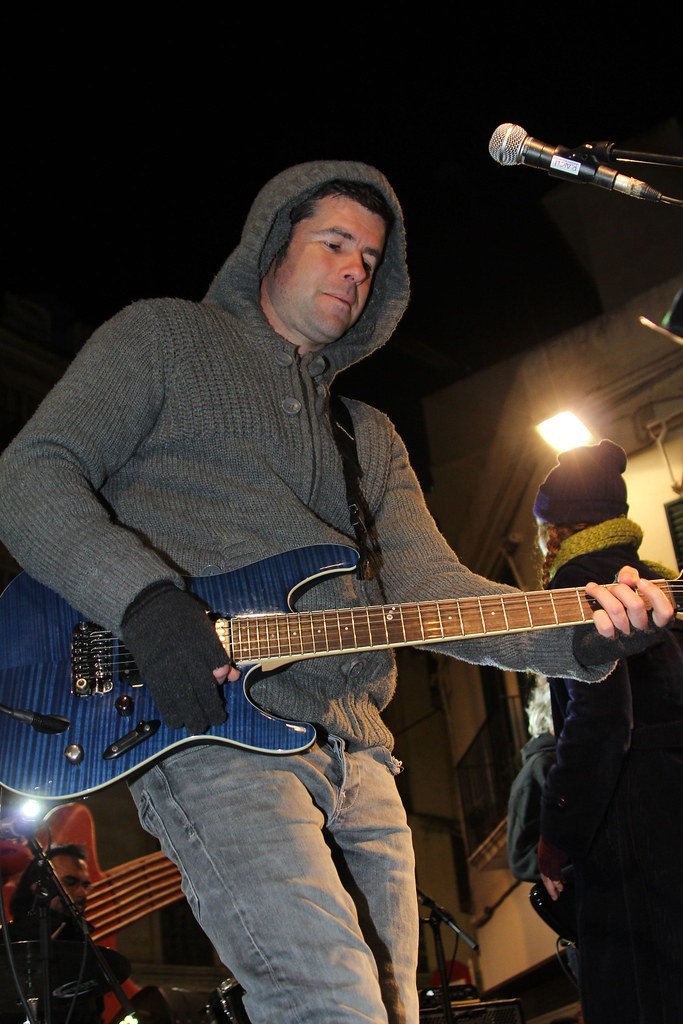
[489,123,663,202]
[0,820,44,841]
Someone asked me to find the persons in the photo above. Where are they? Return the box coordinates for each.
[0,844,105,1024]
[533,440,683,1024]
[0,161,673,1024]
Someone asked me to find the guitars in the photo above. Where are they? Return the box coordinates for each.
[0,541,674,800]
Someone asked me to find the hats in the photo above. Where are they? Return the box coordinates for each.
[535,442,630,525]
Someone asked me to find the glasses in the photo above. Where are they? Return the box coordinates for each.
[60,874,90,890]
